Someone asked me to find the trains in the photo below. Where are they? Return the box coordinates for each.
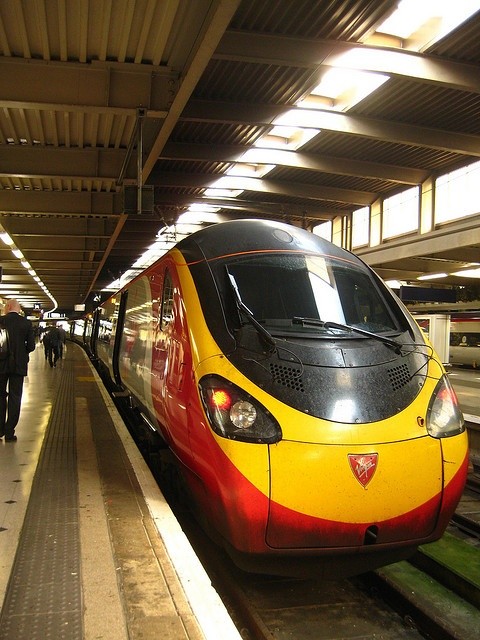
[57,219,469,580]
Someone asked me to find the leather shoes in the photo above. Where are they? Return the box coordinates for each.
[5,436,17,443]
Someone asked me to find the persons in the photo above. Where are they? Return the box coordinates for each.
[0,298,35,442]
[33,322,66,368]
